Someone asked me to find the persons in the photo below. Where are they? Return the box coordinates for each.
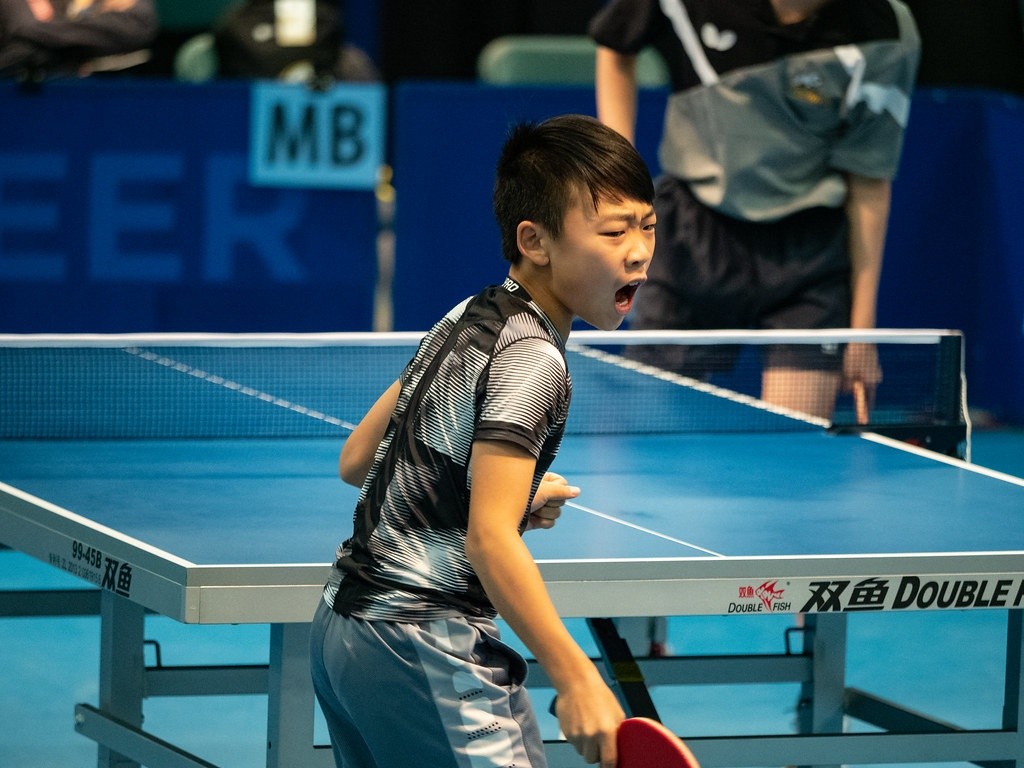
[306,112,657,768]
[0,1,157,77]
[590,0,924,656]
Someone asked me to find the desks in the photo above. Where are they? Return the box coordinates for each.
[0,324,1024,768]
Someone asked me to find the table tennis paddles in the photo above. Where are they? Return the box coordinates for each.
[549,693,702,768]
[853,379,869,425]
